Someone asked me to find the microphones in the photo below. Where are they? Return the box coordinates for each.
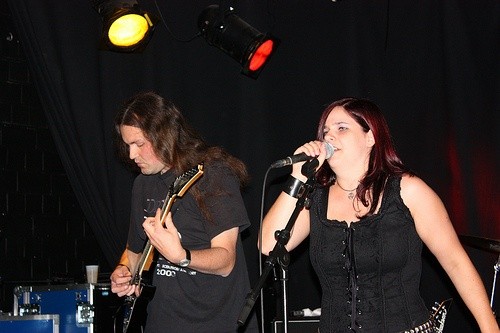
[271,141,334,168]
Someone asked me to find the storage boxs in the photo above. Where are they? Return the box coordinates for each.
[0,314,60,333]
[13,284,111,333]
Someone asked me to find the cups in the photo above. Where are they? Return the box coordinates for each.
[85,265,99,284]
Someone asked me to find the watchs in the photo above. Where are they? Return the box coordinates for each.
[176,248,192,267]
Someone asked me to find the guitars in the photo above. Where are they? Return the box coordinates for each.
[105,162,205,333]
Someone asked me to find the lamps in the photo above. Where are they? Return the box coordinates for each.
[90,0,160,53]
[153,0,282,81]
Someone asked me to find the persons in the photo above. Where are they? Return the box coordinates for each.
[258,95,500,333]
[109,92,259,333]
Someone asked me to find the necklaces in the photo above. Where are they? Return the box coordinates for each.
[334,173,357,199]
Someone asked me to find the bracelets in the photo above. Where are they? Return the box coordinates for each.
[281,174,305,199]
[117,262,130,272]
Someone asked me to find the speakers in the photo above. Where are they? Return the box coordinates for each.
[272,318,320,333]
